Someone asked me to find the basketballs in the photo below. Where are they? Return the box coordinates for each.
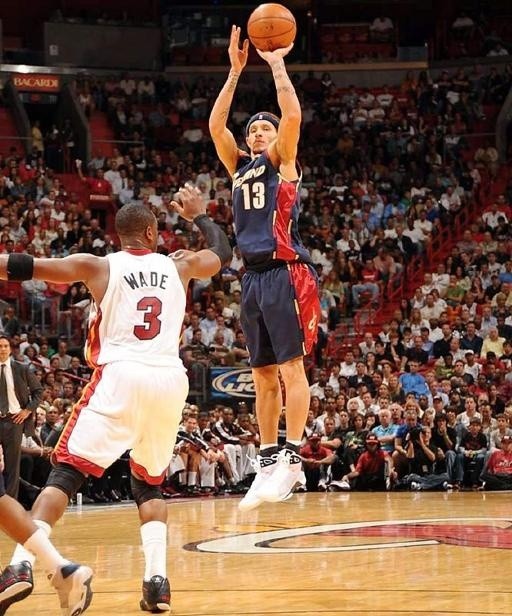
[247,2,297,51]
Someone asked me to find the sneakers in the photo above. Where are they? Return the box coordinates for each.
[47,561,94,616]
[254,449,308,505]
[161,488,180,497]
[330,480,351,492]
[236,454,278,513]
[139,575,171,614]
[0,560,35,616]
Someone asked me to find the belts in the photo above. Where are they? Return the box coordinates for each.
[2,416,15,423]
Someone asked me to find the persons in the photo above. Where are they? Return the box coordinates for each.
[0,336,44,499]
[320,416,344,482]
[0,183,233,569]
[169,271,262,497]
[330,433,398,491]
[299,11,511,180]
[429,413,457,490]
[479,435,512,490]
[300,180,512,424]
[0,306,22,335]
[342,413,368,473]
[453,418,488,490]
[0,445,96,616]
[75,282,93,310]
[162,395,262,497]
[391,411,424,492]
[9,339,36,372]
[489,413,512,448]
[25,333,92,446]
[402,426,450,492]
[19,279,52,327]
[294,432,339,492]
[58,283,78,340]
[1,71,232,258]
[208,24,323,510]
[370,409,400,491]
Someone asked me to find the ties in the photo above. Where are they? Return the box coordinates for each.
[1,362,10,417]
